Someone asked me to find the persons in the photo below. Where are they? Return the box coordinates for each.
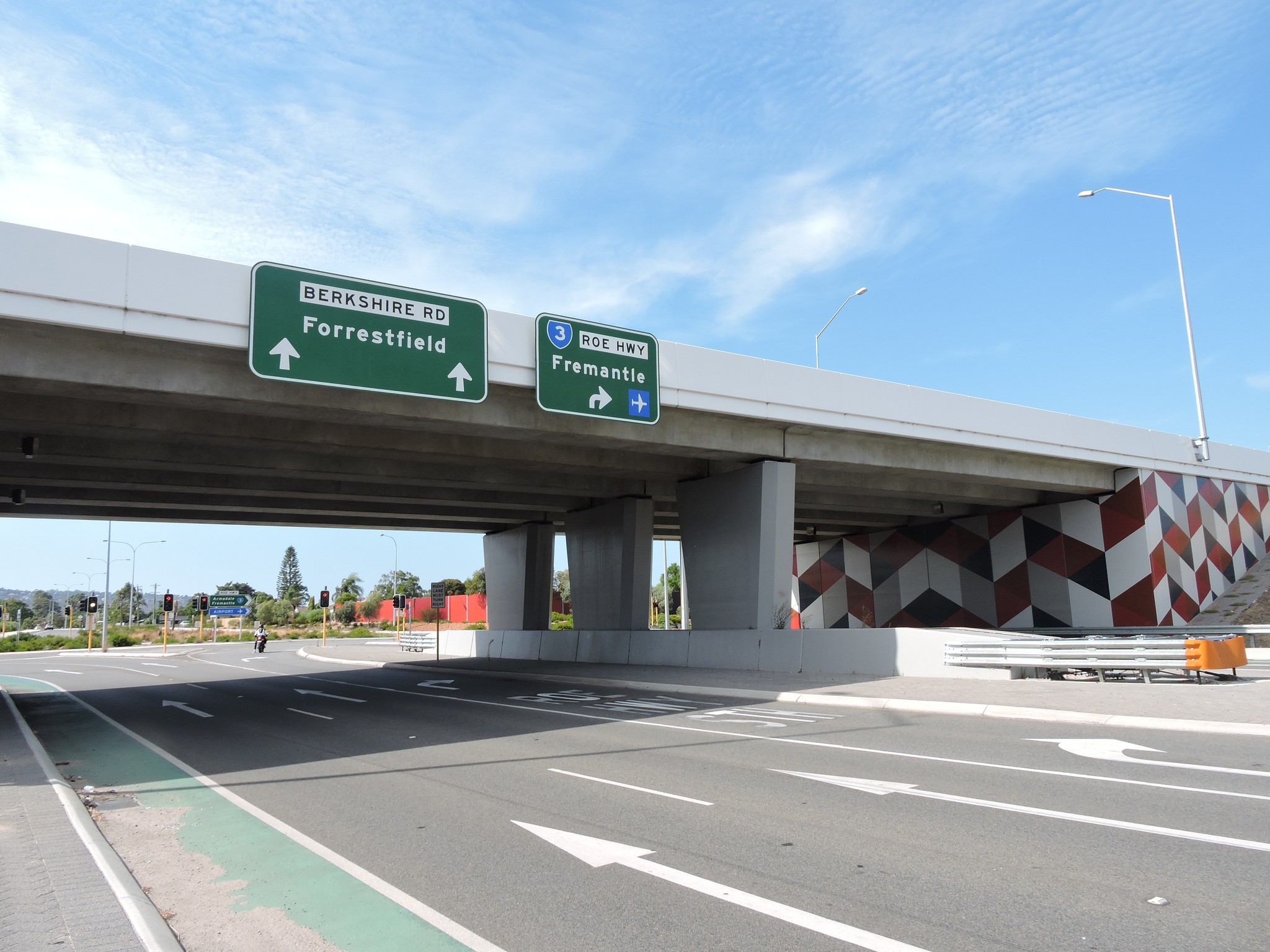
[251,624,269,653]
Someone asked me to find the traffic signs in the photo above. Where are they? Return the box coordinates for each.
[209,594,253,608]
[534,312,663,424]
[209,608,252,615]
[399,595,406,607]
[247,262,489,403]
[192,596,199,611]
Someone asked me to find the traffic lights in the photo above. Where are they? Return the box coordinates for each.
[86,597,98,614]
[320,590,332,609]
[65,605,71,617]
[80,598,88,613]
[392,594,400,609]
[406,604,409,610]
[162,594,174,612]
[0,605,3,618]
[199,596,210,611]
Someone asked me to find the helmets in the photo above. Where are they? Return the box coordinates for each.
[259,624,264,628]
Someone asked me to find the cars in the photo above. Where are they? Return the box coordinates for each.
[180,620,191,626]
[45,624,55,629]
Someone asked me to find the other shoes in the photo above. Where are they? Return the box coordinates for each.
[264,644,266,648]
[252,651,255,653]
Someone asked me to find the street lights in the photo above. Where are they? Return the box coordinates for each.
[73,572,108,597]
[102,539,167,629]
[54,584,84,628]
[52,590,61,625]
[817,287,868,371]
[1079,187,1212,464]
[380,533,397,626]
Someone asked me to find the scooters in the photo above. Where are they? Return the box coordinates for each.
[255,635,268,653]
